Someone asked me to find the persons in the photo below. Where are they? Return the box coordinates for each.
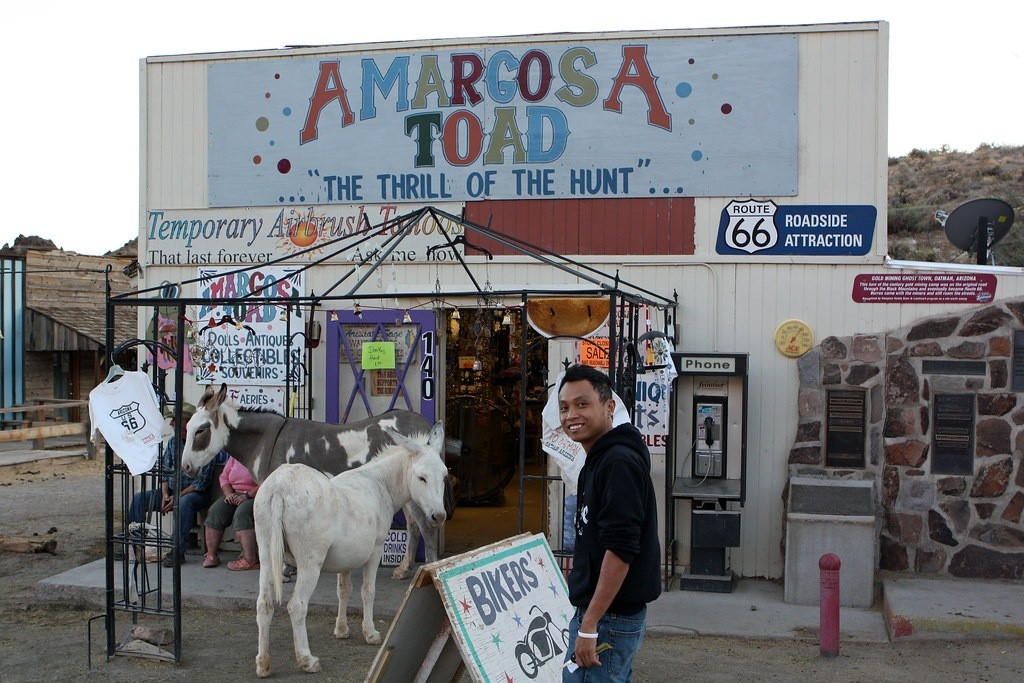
[114,402,215,568]
[203,453,261,570]
[556,366,662,683]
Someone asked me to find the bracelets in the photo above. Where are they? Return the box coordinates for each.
[577,629,600,639]
[242,490,251,500]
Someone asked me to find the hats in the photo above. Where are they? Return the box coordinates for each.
[169,402,196,426]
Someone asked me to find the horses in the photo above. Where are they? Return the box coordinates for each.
[254,420,448,678]
[180,382,444,582]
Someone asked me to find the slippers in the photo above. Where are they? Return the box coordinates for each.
[204,552,220,567]
[228,560,261,571]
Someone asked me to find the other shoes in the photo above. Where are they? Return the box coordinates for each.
[114,549,124,561]
[162,555,185,568]
